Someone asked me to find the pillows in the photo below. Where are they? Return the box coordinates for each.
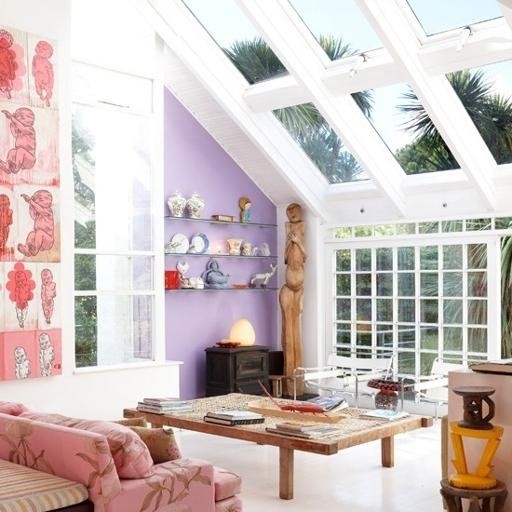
[112,415,180,461]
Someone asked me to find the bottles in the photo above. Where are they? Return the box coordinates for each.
[167,191,205,219]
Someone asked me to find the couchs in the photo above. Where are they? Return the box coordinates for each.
[0,400,241,512]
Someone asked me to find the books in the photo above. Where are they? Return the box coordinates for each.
[136,395,409,440]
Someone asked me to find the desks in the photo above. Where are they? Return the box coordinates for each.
[440,366,511,512]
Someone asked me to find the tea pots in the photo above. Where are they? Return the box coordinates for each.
[243,241,270,257]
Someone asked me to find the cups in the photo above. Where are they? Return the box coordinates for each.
[165,271,179,290]
[227,238,242,256]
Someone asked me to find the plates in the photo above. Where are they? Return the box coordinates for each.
[172,232,210,255]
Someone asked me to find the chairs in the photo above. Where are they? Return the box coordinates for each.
[290,353,489,422]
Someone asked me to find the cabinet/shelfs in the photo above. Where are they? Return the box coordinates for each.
[204,344,270,396]
[164,215,280,292]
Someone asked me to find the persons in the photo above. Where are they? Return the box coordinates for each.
[0,30,57,380]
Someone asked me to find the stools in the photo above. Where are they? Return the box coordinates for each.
[0,459,95,512]
